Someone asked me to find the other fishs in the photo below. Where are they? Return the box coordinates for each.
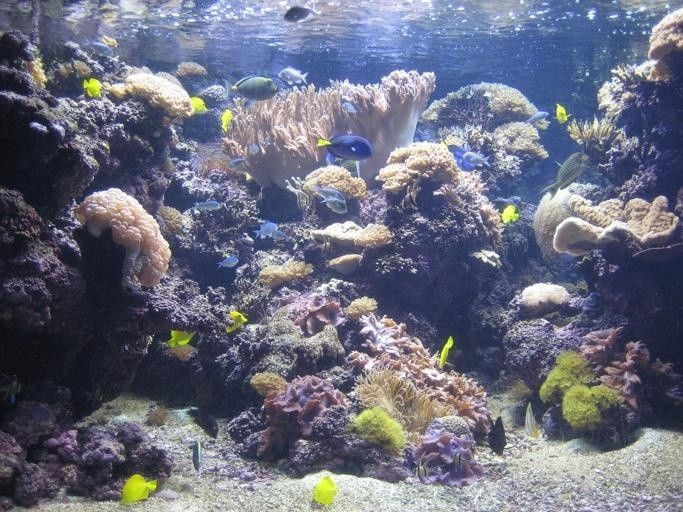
[82,34,117,99]
[314,473,338,509]
[415,401,548,485]
[445,140,495,174]
[119,472,160,508]
[496,204,520,228]
[525,102,591,202]
[316,99,376,218]
[194,201,286,269]
[438,335,456,370]
[400,184,424,214]
[179,407,220,474]
[166,312,253,350]
[184,66,313,166]
[0,371,24,406]
[284,2,320,23]
